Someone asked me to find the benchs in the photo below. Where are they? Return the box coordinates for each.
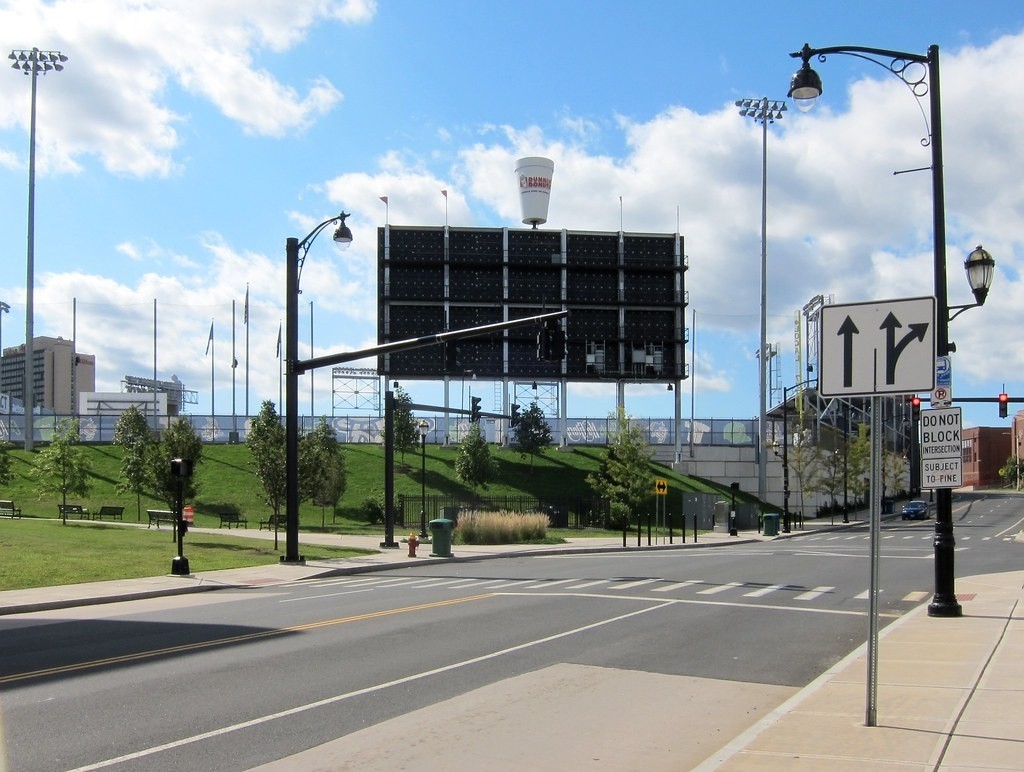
[147,510,185,530]
[58,504,89,519]
[260,515,287,531]
[92,505,125,520]
[0,500,21,519]
[219,513,248,529]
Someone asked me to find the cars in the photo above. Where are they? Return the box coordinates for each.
[901,501,930,521]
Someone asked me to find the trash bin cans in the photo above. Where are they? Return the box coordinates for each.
[429,519,455,557]
[763,514,781,536]
[885,501,893,513]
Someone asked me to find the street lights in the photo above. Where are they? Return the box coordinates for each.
[0,301,10,360]
[277,211,354,565]
[785,41,997,617]
[782,377,818,533]
[8,46,69,453]
[734,94,791,505]
[418,418,431,537]
[843,400,870,523]
[755,343,777,410]
[881,412,911,514]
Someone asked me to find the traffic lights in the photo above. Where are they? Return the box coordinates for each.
[510,403,520,427]
[998,393,1007,418]
[912,397,921,421]
[471,395,482,421]
[171,457,194,478]
[538,326,553,360]
[552,331,568,360]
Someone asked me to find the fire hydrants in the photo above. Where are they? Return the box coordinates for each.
[408,531,420,557]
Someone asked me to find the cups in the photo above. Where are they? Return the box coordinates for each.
[514,157,554,225]
